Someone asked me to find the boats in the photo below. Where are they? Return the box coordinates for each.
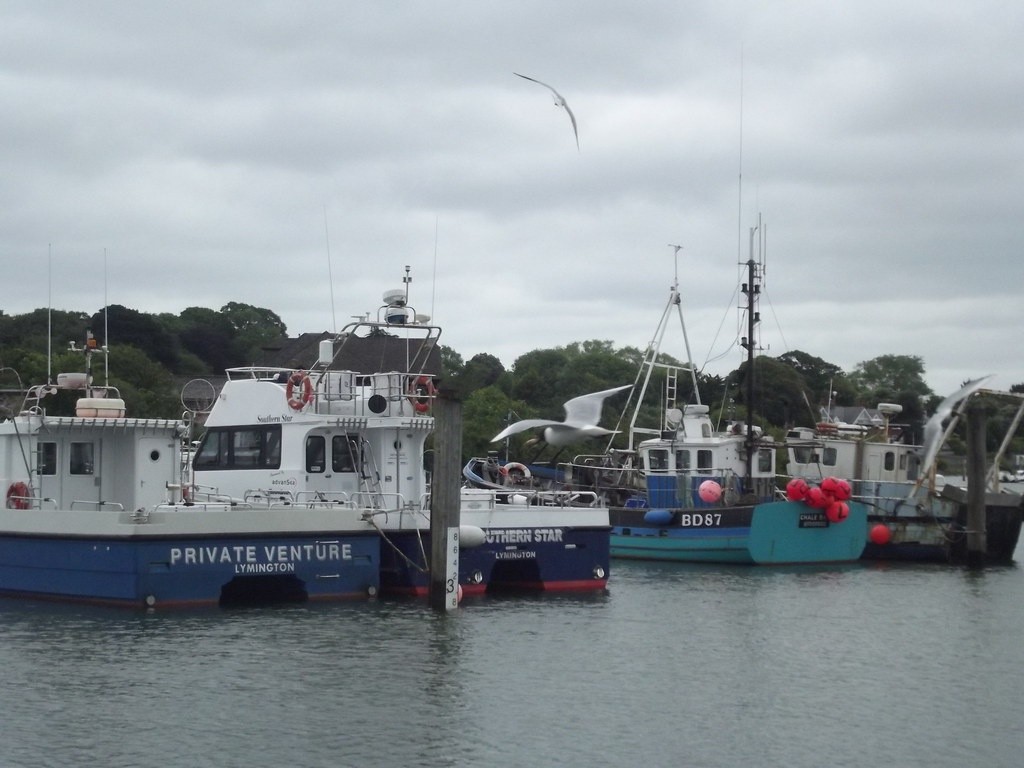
[574,374,1024,565]
[460,52,869,566]
[1,199,613,607]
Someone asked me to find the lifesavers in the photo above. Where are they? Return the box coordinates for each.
[817,423,868,436]
[410,376,436,411]
[286,373,311,410]
[6,482,29,509]
[502,462,531,484]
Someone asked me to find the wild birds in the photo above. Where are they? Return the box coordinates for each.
[489,384,635,447]
[918,374,994,480]
[514,72,579,149]
